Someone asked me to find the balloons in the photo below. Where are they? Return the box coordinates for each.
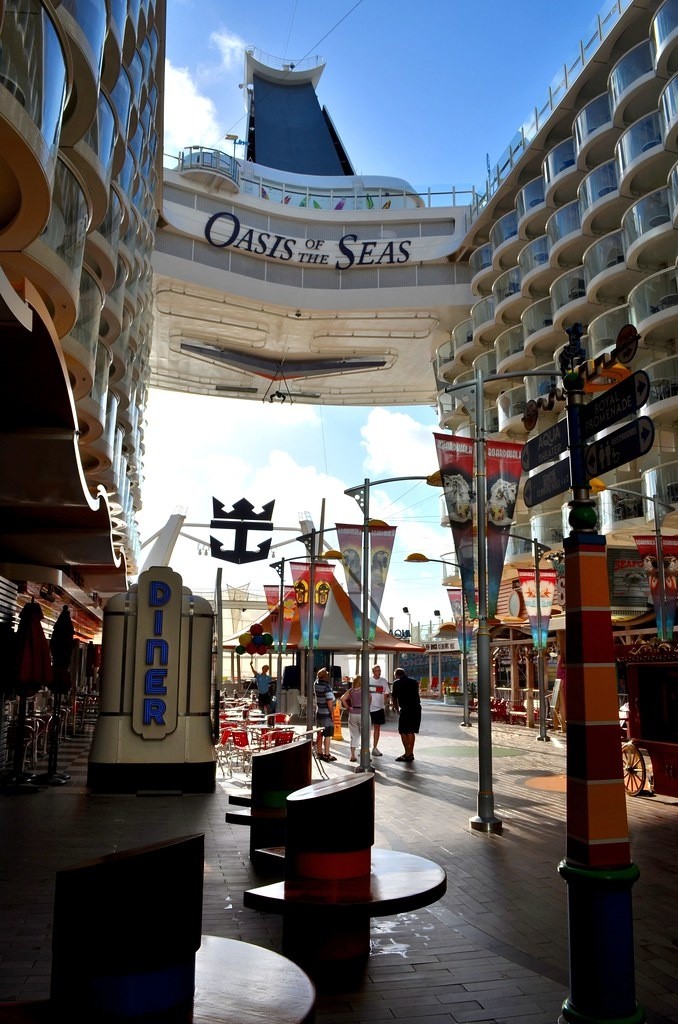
[236,624,273,655]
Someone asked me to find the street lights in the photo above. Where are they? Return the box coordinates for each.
[307,518,388,741]
[402,553,472,728]
[276,550,342,717]
[472,366,584,830]
[589,477,669,640]
[475,527,550,742]
[403,607,412,644]
[434,610,444,700]
[358,466,445,771]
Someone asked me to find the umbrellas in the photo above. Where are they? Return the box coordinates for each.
[33,605,73,786]
[71,640,96,737]
[0,602,52,784]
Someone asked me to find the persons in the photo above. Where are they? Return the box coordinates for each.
[369,666,390,756]
[313,667,337,761]
[250,662,282,713]
[392,668,422,761]
[340,676,371,762]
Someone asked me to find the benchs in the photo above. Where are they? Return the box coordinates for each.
[1,739,448,1024]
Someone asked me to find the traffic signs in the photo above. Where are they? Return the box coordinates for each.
[520,364,653,510]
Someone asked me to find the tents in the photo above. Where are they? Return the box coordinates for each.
[220,565,425,711]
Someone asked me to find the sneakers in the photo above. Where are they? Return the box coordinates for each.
[372,748,382,756]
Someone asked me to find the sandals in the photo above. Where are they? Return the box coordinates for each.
[317,753,323,759]
[322,754,337,760]
[395,754,414,761]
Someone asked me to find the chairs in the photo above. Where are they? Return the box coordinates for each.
[16,705,71,771]
[211,696,331,781]
[467,697,529,724]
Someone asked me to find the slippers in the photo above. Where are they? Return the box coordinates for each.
[350,758,357,762]
